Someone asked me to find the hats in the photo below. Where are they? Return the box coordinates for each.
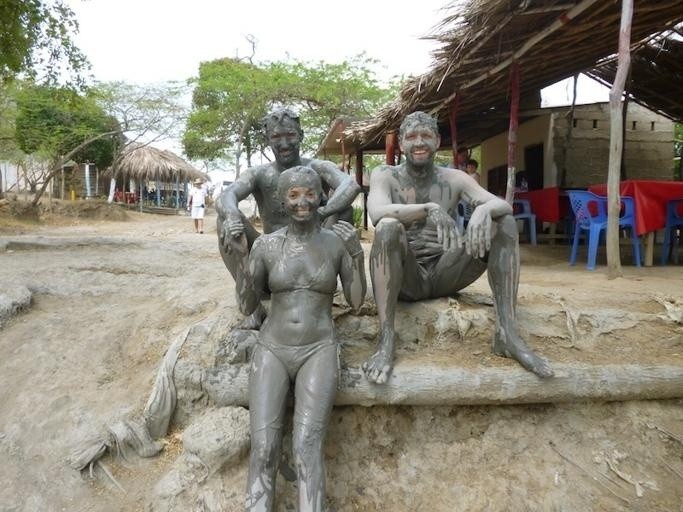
[193,179,203,184]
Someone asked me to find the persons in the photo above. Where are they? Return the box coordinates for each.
[214,109,361,330]
[361,111,555,386]
[220,166,367,511]
[185,176,210,235]
[447,146,469,172]
[463,155,480,186]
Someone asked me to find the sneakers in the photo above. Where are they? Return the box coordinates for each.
[200,232,203,234]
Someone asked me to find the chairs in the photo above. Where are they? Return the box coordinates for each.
[564,179,683,270]
[455,199,475,235]
[513,199,537,247]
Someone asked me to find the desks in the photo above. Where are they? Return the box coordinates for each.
[514,186,587,246]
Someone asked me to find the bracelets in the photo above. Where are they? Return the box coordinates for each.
[350,250,363,257]
